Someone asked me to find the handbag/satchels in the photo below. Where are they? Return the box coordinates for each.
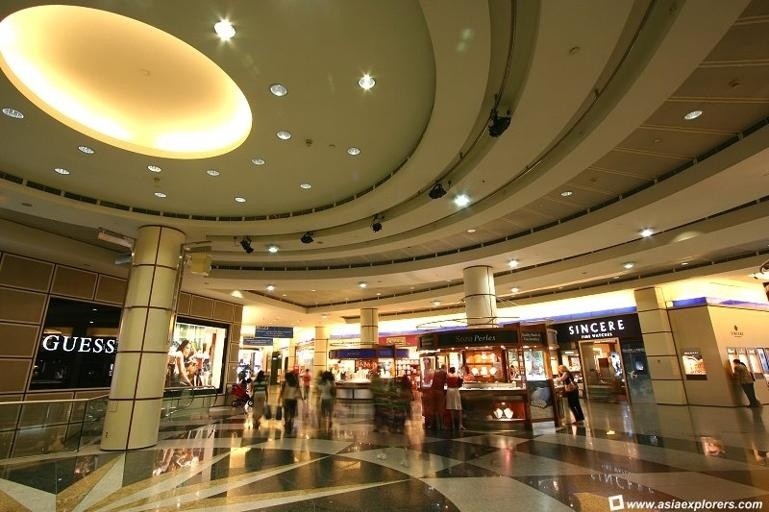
[329,386,337,395]
[264,405,271,419]
[276,406,282,420]
[564,381,577,392]
[457,376,463,387]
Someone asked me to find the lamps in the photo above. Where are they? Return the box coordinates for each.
[240,236,254,254]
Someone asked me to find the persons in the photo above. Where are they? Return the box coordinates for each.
[557,365,585,425]
[366,366,415,435]
[165,336,212,387]
[421,359,478,431]
[242,364,348,436]
[732,359,762,408]
[509,364,519,380]
